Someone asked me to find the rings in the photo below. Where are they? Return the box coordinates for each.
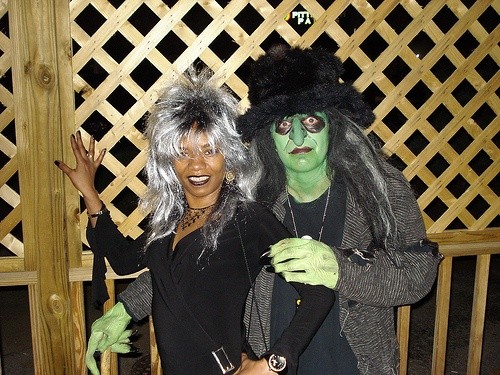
[87,154,93,158]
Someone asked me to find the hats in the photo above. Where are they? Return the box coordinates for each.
[234,44,376,144]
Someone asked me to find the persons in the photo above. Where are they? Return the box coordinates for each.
[85,49,444,375]
[53,87,336,375]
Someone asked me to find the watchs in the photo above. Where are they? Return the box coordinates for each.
[265,354,289,375]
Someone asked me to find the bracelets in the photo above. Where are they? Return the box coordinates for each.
[87,208,108,219]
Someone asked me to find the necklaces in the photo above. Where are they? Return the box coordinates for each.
[180,203,215,232]
[285,185,331,241]
